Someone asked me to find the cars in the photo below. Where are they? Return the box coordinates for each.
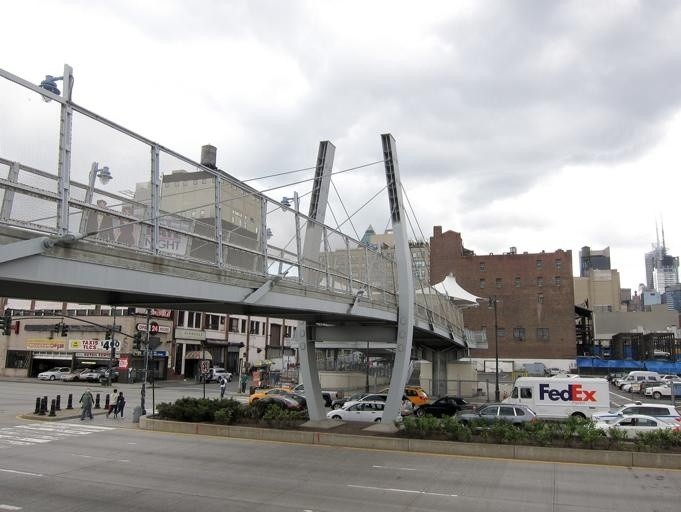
[644,348,671,359]
[36,364,119,383]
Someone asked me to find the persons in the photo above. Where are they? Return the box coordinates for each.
[221,376,228,398]
[104,370,109,386]
[87,200,113,240]
[106,389,118,419]
[241,374,249,393]
[80,390,94,421]
[118,206,135,248]
[114,391,127,419]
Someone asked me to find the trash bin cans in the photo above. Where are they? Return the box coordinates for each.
[250,386,256,395]
[477,388,483,396]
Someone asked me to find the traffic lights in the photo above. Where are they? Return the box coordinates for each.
[132,331,141,350]
[1,315,12,336]
[53,323,59,333]
[104,328,111,340]
[60,324,68,336]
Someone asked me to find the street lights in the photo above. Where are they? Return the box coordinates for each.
[251,226,272,273]
[264,334,272,360]
[487,293,501,403]
[38,62,72,238]
[278,190,304,286]
[76,162,113,236]
[244,329,255,376]
[280,334,289,376]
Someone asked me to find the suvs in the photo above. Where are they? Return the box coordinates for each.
[205,367,233,384]
